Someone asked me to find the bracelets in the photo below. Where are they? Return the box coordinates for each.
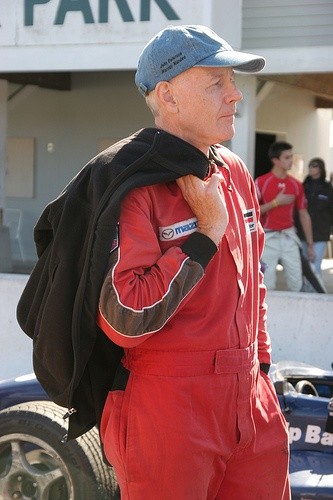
[273,199,277,207]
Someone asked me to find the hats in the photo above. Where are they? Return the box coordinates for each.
[133,23,266,97]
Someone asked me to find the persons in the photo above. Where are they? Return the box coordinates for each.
[83,24,291,500]
[253,141,316,292]
[292,158,333,294]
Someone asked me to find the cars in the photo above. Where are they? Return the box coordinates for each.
[0,361,333,500]
